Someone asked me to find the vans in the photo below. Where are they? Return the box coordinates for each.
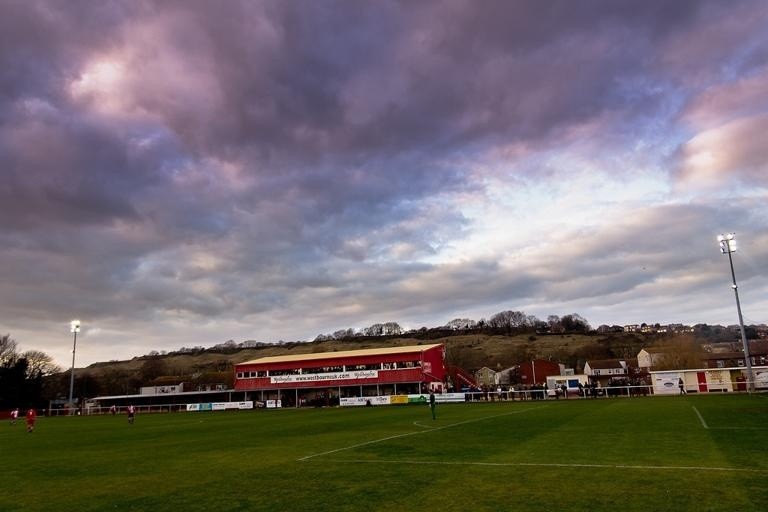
[461,387,480,400]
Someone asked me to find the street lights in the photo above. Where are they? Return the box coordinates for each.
[718,231,756,392]
[67,320,83,405]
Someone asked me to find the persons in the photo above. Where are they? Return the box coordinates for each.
[27,408,35,433]
[429,390,436,421]
[465,378,647,403]
[10,408,20,425]
[110,404,116,416]
[127,401,136,424]
[679,378,686,395]
